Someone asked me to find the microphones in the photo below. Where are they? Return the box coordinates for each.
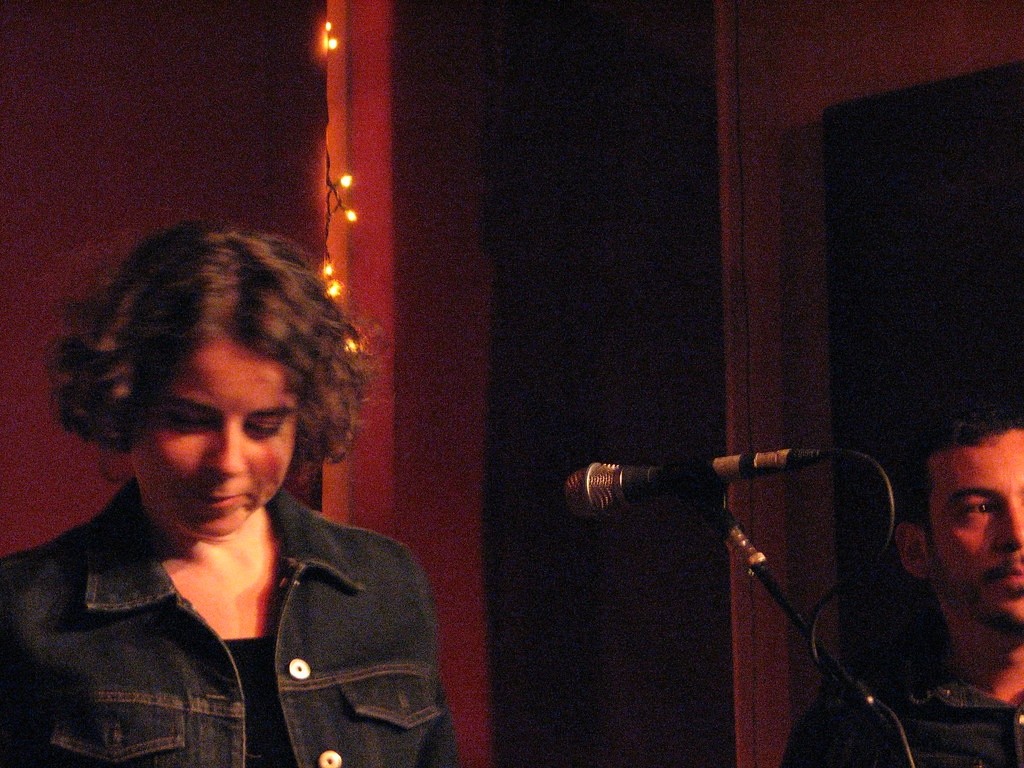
[567,448,825,519]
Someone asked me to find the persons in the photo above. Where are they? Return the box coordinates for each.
[0,221,464,768]
[779,387,1024,768]
